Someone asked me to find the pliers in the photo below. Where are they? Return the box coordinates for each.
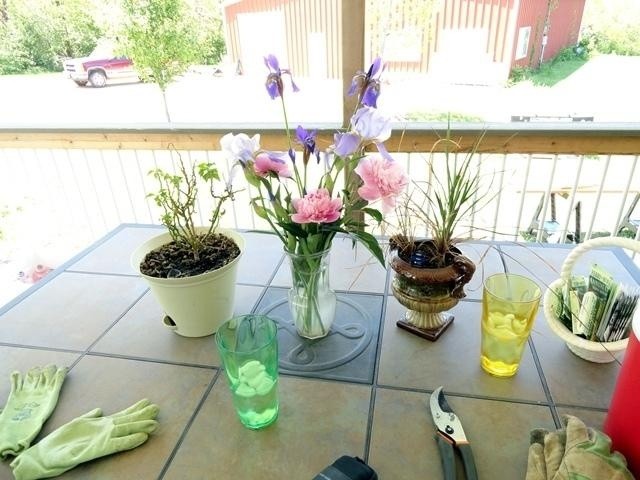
[430,386,479,480]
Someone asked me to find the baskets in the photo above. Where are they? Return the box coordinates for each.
[543,237,640,364]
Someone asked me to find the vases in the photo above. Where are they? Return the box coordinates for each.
[280,242,335,339]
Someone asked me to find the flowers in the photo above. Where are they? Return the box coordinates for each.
[223,52,408,335]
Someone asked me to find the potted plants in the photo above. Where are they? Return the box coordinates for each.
[369,116,520,341]
[131,142,247,338]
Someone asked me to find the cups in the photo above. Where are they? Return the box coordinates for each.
[478,268,543,382]
[215,312,290,432]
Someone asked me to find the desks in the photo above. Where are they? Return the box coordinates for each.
[0,222,639,480]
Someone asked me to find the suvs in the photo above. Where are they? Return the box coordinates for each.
[63,38,155,88]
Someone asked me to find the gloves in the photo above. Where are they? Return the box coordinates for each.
[10,399,160,480]
[0,365,66,461]
[526,414,636,480]
